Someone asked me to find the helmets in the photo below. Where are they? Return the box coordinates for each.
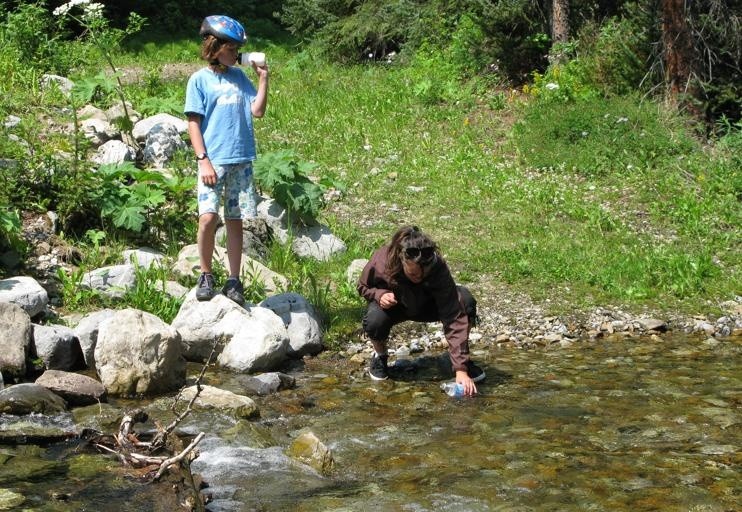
[199,15,247,46]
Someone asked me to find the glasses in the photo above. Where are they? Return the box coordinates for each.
[405,247,435,257]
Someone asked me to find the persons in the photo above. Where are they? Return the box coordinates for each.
[182,11,270,304]
[358,224,485,399]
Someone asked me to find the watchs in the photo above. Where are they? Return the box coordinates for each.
[195,150,208,161]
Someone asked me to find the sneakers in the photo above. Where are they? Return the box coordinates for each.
[196,272,216,301]
[369,352,389,381]
[222,278,245,306]
[465,360,485,382]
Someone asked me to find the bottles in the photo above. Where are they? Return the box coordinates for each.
[236,52,266,68]
[439,383,464,397]
